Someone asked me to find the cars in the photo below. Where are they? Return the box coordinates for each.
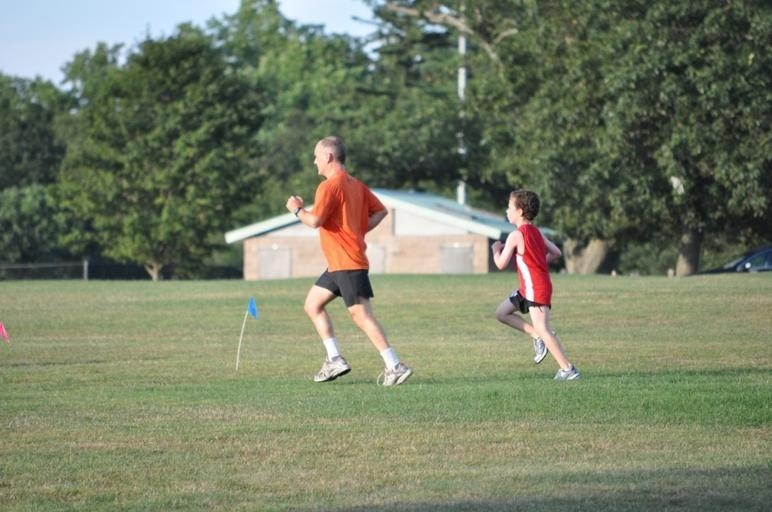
[685,245,772,275]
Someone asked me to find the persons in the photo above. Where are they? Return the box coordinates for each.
[488,187,581,381]
[279,130,416,387]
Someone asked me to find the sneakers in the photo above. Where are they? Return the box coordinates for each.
[313,355,351,382]
[552,365,581,380]
[382,362,413,385]
[533,327,556,365]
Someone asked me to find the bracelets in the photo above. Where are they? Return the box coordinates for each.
[293,205,303,219]
